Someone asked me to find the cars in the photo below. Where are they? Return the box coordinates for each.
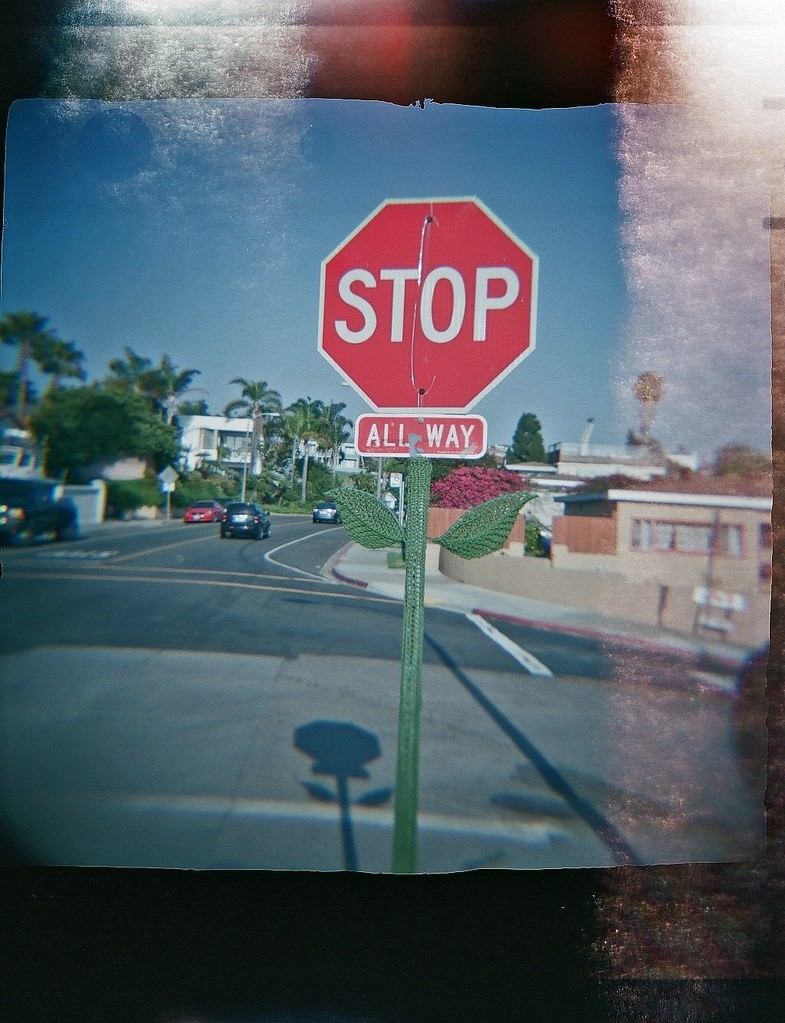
[220,503,272,539]
[0,477,76,543]
[313,502,341,524]
[183,499,226,523]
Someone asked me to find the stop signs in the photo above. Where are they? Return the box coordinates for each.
[316,195,538,414]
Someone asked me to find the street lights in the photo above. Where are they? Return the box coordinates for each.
[241,412,281,503]
